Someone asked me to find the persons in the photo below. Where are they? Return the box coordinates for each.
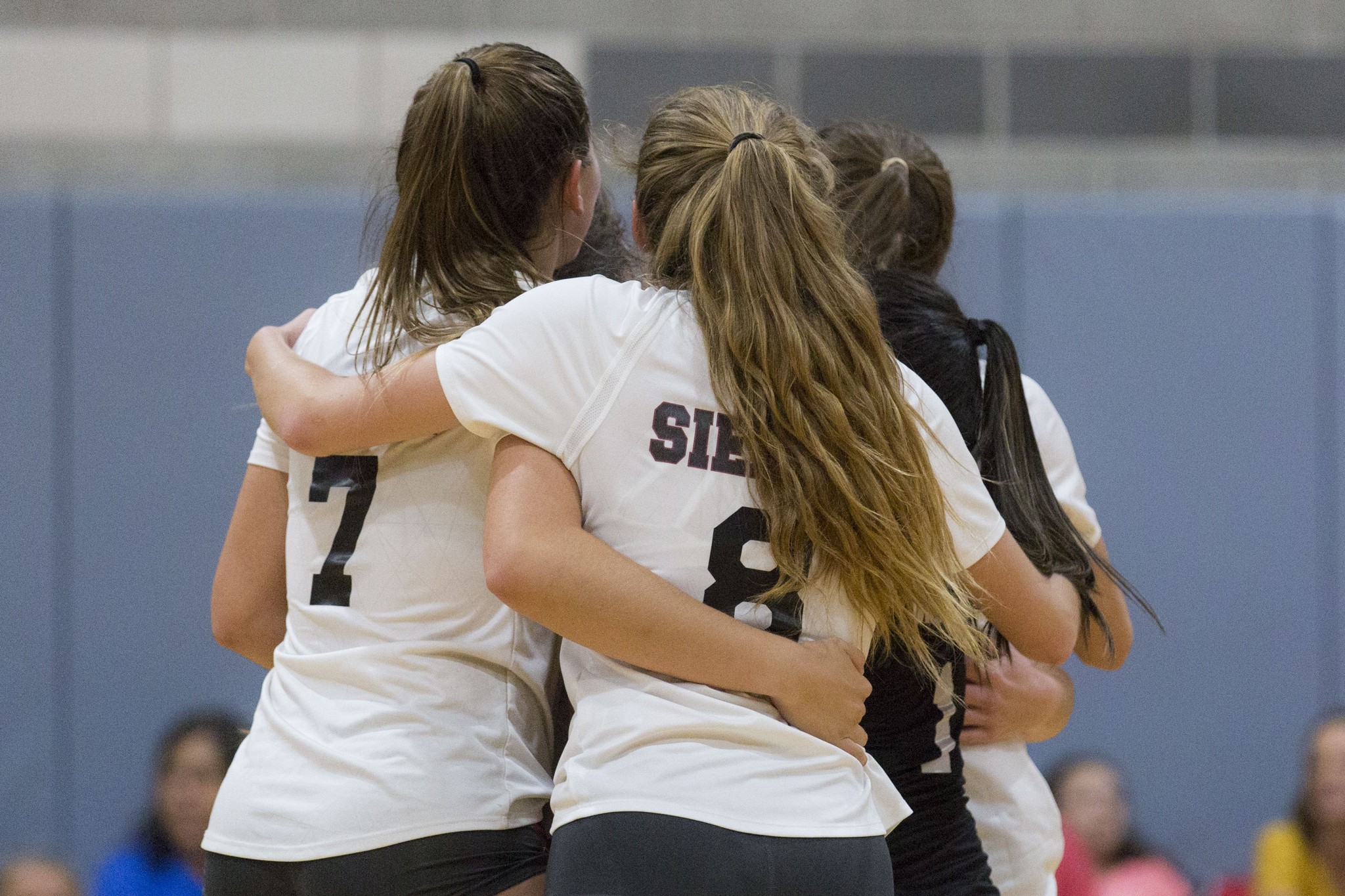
[0,44,1193,896]
[1248,707,1345,896]
